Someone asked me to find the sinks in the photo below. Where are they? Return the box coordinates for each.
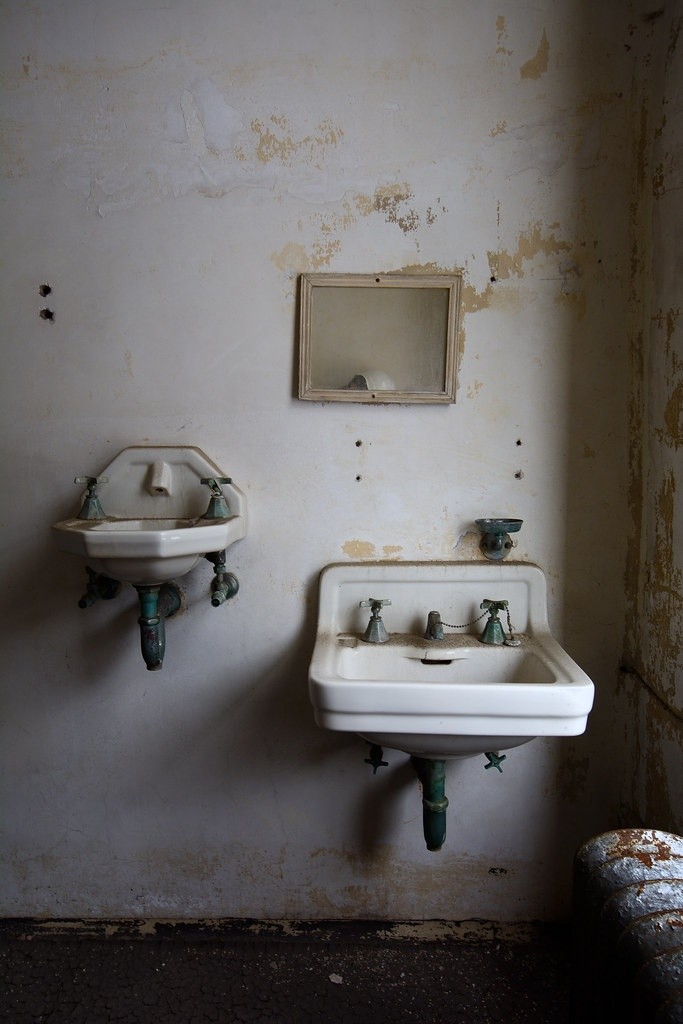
[53,445,243,583]
[309,559,594,758]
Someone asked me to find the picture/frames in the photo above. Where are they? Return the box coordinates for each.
[294,273,460,404]
